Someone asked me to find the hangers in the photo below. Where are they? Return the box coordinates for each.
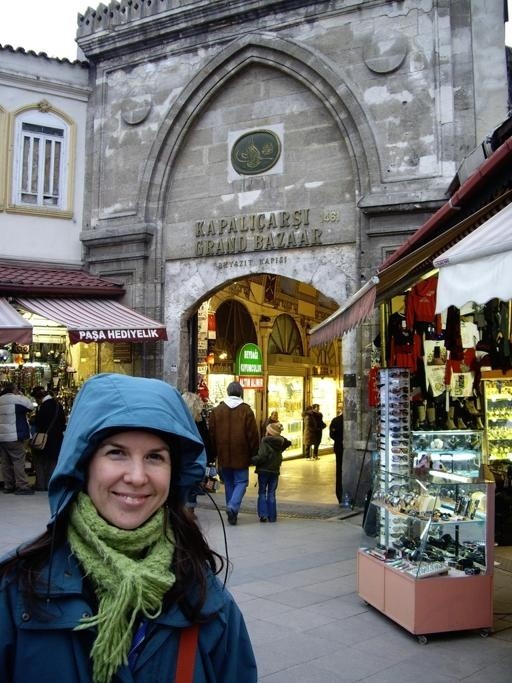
[458,314,472,323]
[495,313,505,344]
[398,297,406,317]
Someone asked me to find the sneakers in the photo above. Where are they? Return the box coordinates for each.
[258,515,266,522]
[306,458,314,461]
[313,457,320,460]
[226,509,237,524]
[4,489,15,493]
[15,489,35,495]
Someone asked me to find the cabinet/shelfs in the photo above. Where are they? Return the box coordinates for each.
[353,431,495,638]
[485,372,510,467]
[374,367,412,550]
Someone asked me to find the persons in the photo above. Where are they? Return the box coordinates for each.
[301,406,312,444]
[1,371,260,683]
[261,410,279,438]
[28,384,67,492]
[251,421,292,523]
[328,412,344,503]
[180,391,215,521]
[1,378,34,494]
[206,379,261,525]
[305,404,326,461]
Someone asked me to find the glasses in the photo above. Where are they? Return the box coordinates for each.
[412,484,488,579]
[372,371,412,569]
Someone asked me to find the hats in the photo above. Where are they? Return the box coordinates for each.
[266,423,284,436]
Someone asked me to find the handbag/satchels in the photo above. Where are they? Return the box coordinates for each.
[29,432,48,449]
